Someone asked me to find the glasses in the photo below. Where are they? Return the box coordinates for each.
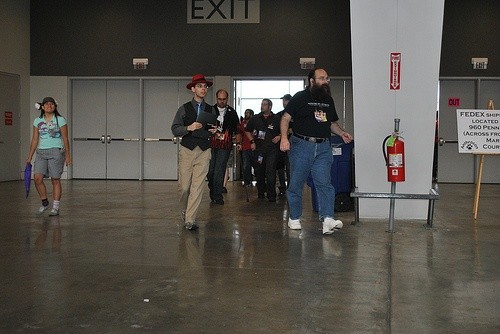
[313,77,330,81]
[196,84,209,89]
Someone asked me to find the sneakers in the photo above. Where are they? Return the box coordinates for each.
[323,217,343,235]
[288,219,302,230]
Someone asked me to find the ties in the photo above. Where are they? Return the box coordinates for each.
[196,102,202,122]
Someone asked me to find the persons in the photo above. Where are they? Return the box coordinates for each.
[171,73,219,230]
[275,93,294,196]
[206,89,242,206]
[243,98,281,202]
[27,96,72,216]
[238,108,256,187]
[279,68,354,235]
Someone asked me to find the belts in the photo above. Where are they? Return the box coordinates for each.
[293,133,327,143]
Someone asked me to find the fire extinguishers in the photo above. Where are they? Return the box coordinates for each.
[383,130,407,183]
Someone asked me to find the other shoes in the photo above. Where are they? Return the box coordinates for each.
[258,193,264,200]
[277,191,286,197]
[48,208,59,216]
[181,210,186,220]
[184,223,199,230]
[214,199,225,205]
[222,187,227,193]
[35,203,51,216]
[268,197,276,202]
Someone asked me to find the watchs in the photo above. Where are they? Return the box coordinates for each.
[236,141,243,144]
[250,139,256,144]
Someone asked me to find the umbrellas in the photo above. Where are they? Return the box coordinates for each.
[24,162,32,198]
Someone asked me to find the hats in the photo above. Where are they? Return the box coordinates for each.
[42,97,55,103]
[186,74,213,90]
[280,94,293,100]
